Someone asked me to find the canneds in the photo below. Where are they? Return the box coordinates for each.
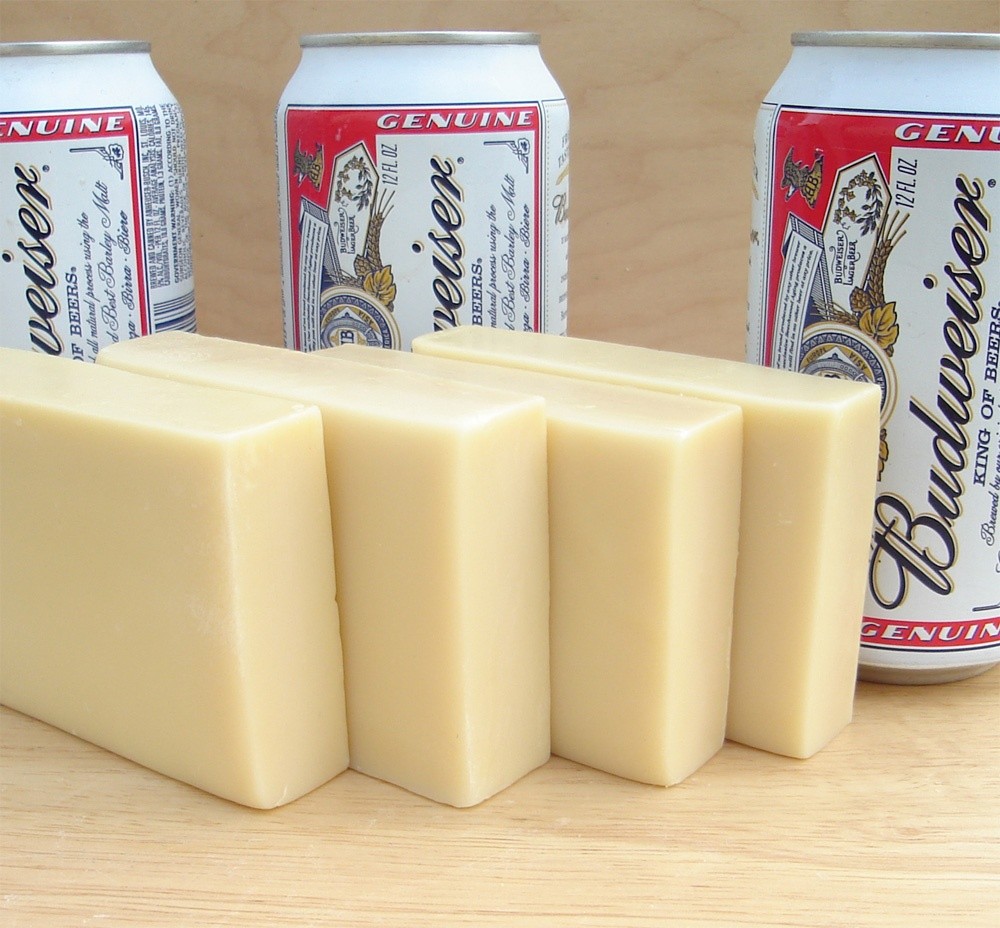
[0,40,197,363]
[748,30,999,687]
[271,32,569,359]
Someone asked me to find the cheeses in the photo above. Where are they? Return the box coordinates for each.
[0,325,881,808]
